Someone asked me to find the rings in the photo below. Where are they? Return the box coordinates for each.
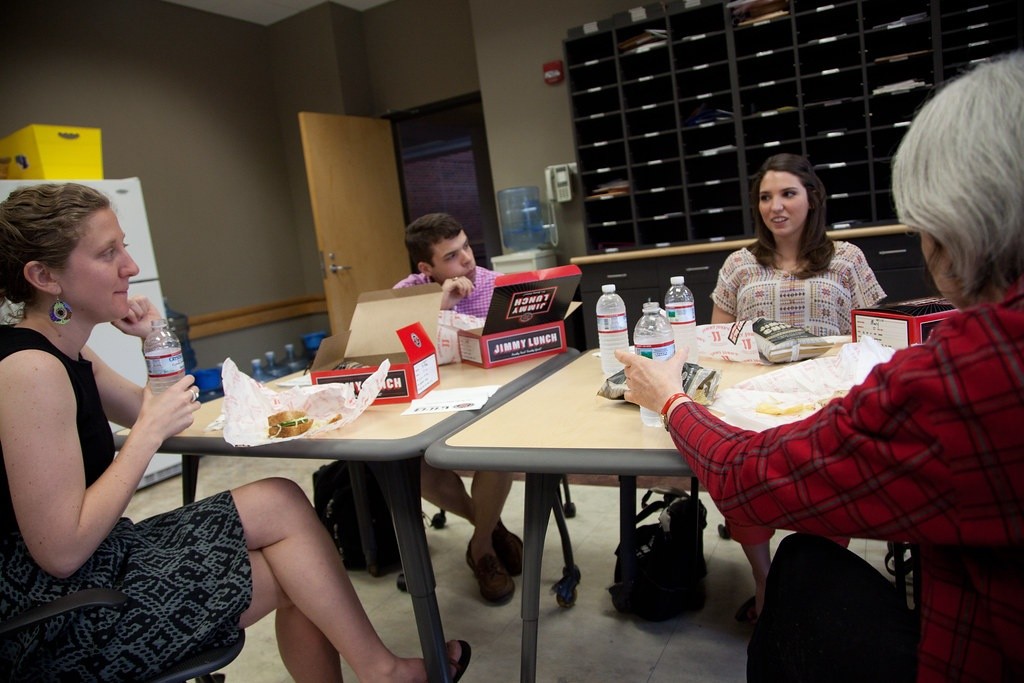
[188,389,197,403]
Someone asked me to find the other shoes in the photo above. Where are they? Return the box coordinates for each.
[466,518,523,603]
[734,596,756,625]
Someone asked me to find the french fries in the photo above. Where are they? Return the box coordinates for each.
[756,405,805,414]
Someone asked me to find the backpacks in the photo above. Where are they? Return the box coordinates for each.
[312,460,401,571]
[607,488,707,623]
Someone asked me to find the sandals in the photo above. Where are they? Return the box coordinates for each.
[447,639,472,683]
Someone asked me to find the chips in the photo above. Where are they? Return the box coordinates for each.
[694,389,712,405]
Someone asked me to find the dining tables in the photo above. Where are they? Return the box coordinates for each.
[424,345,904,683]
[111,349,580,683]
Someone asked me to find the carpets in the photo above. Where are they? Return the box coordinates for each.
[453,469,708,491]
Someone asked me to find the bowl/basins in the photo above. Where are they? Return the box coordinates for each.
[190,368,222,394]
[302,331,327,350]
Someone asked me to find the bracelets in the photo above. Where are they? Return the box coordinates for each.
[662,392,695,431]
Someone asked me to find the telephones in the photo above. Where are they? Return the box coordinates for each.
[544,163,575,204]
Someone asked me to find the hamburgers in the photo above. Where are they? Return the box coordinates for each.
[268,410,313,438]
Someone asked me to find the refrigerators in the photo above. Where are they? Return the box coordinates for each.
[0,176,183,493]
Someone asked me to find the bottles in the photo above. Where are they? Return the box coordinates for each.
[633,302,675,428]
[595,284,630,380]
[664,275,699,364]
[163,296,197,374]
[143,318,186,400]
[497,186,547,253]
[250,342,311,386]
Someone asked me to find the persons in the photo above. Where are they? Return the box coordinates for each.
[710,154,888,613]
[392,214,525,604]
[613,50,1024,682]
[0,181,470,683]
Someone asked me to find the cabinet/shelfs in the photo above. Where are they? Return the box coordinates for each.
[562,0,1024,256]
[570,224,942,349]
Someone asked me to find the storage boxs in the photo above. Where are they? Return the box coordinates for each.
[457,264,582,369]
[851,294,955,349]
[0,124,103,180]
[311,283,443,406]
